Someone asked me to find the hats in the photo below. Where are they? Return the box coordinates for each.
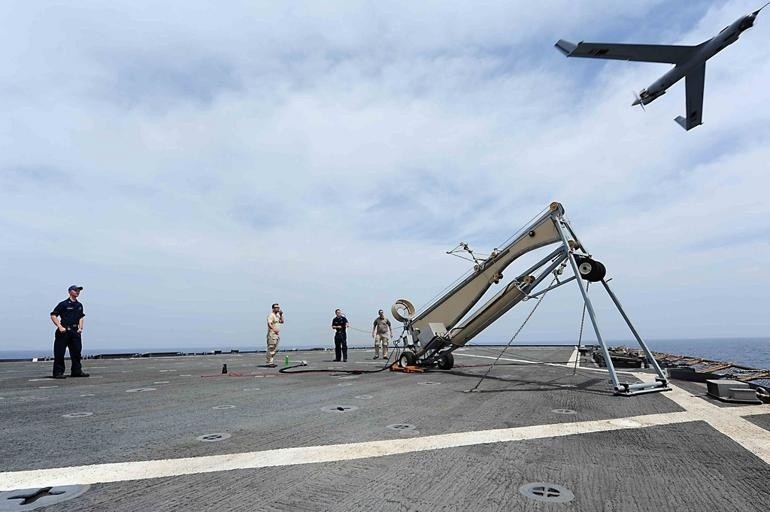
[69,285,83,292]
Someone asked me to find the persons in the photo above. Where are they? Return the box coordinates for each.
[372,309,392,360]
[264,303,285,368]
[50,285,90,379]
[331,309,351,362]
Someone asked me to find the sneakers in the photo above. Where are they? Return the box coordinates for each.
[333,360,347,362]
[373,355,389,359]
[71,372,89,377]
[266,364,277,367]
[54,373,66,378]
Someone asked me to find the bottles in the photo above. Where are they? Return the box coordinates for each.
[285,356,288,367]
[222,364,228,374]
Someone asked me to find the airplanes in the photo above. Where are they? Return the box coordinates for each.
[553,0,770,132]
